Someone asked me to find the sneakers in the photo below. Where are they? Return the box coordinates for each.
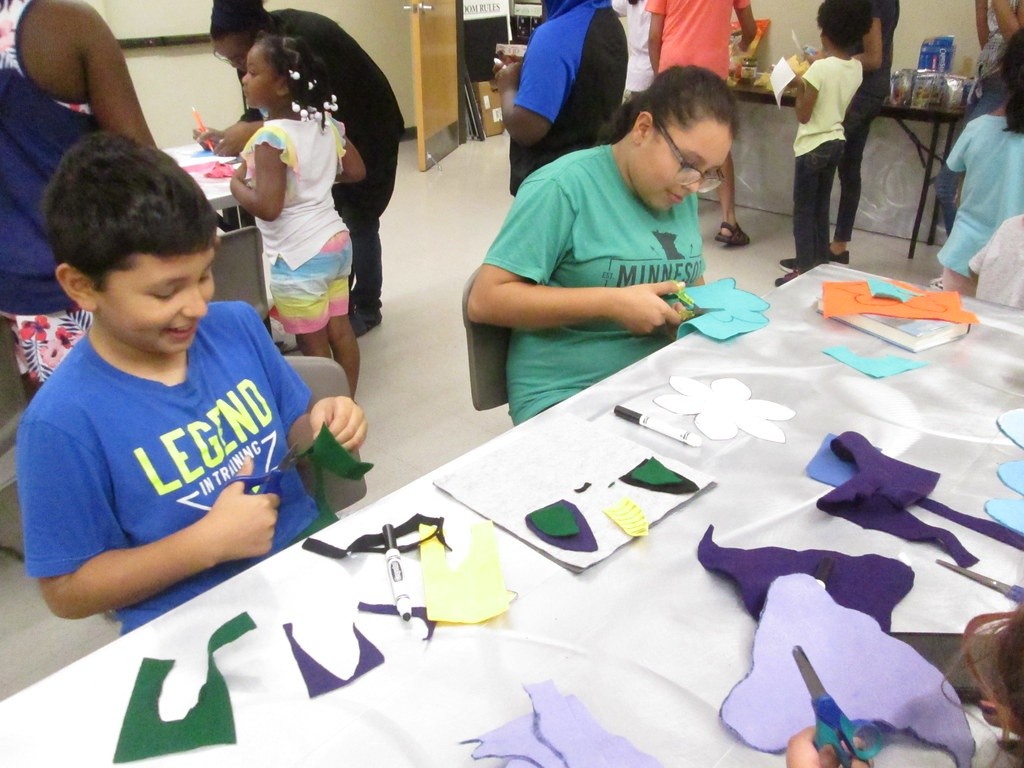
[779,258,801,272]
[827,243,850,267]
[350,299,382,337]
[775,269,803,288]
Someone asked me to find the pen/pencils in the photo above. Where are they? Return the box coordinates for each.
[191,106,214,152]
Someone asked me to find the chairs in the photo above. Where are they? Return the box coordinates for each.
[279,356,368,514]
[461,264,513,413]
[207,226,273,340]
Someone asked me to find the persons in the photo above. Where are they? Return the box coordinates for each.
[937,0,1024,309]
[0,0,157,391]
[780,0,901,273]
[786,601,1024,768]
[492,0,757,245]
[16,132,368,637]
[775,0,873,287]
[230,32,366,403]
[467,64,740,428]
[192,0,404,338]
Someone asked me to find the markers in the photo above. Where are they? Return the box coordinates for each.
[382,524,412,621]
[614,405,703,448]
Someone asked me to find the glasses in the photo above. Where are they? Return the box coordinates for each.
[659,123,725,194]
[213,50,247,66]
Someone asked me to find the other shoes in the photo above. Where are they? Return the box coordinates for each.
[928,276,943,290]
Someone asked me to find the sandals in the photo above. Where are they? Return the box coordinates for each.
[715,221,750,245]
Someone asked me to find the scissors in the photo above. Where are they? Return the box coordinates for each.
[936,559,1024,605]
[220,442,308,497]
[791,645,885,768]
[668,280,726,321]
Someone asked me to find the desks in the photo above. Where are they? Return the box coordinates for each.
[156,142,257,229]
[0,265,1023,767]
[735,78,964,261]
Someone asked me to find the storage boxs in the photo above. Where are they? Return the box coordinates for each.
[471,81,509,137]
